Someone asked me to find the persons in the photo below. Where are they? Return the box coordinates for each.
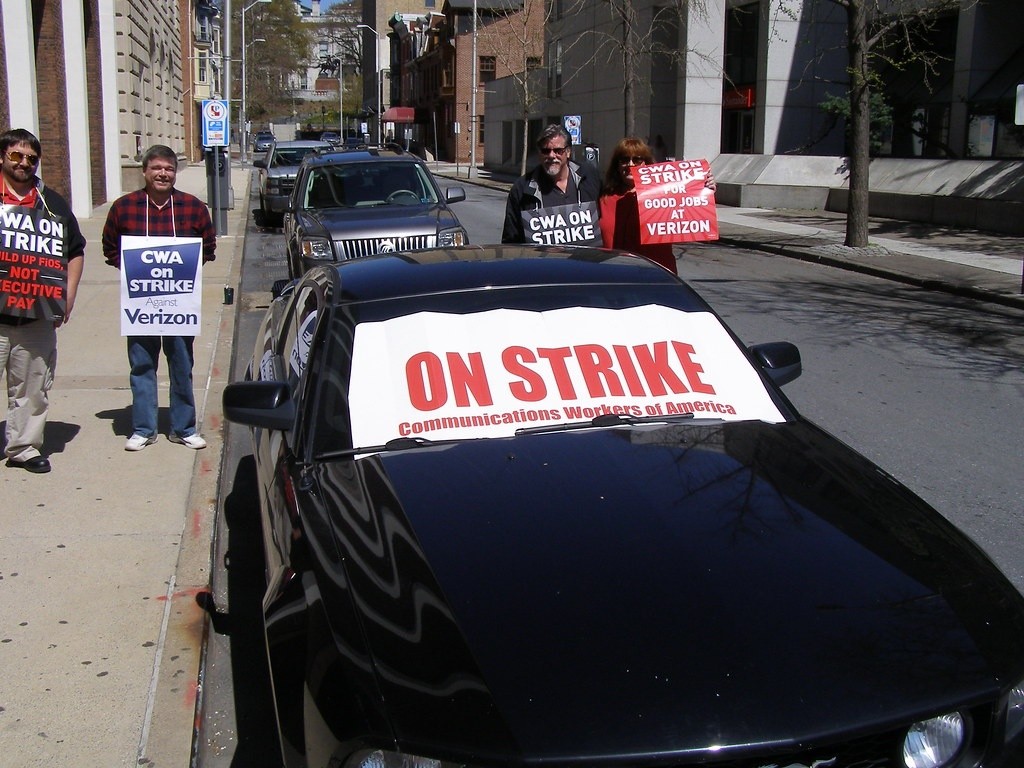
[381,129,385,148]
[598,137,678,274]
[231,127,236,142]
[304,123,313,140]
[501,126,605,247]
[0,128,87,474]
[102,144,217,451]
[253,133,257,137]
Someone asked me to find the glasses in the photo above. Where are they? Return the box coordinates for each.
[3,151,39,166]
[618,156,646,166]
[540,145,568,155]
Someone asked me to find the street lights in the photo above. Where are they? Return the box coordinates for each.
[241,0,272,163]
[356,25,381,143]
[334,54,345,146]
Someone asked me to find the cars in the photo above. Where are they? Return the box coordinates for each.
[221,242,1024,768]
[255,132,276,151]
[252,142,343,224]
[320,132,339,147]
[285,146,470,283]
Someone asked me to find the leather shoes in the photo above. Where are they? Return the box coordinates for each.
[7,455,51,472]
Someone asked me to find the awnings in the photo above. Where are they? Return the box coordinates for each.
[381,107,414,123]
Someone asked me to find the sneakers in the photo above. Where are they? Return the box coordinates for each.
[125,432,158,450]
[168,433,207,449]
